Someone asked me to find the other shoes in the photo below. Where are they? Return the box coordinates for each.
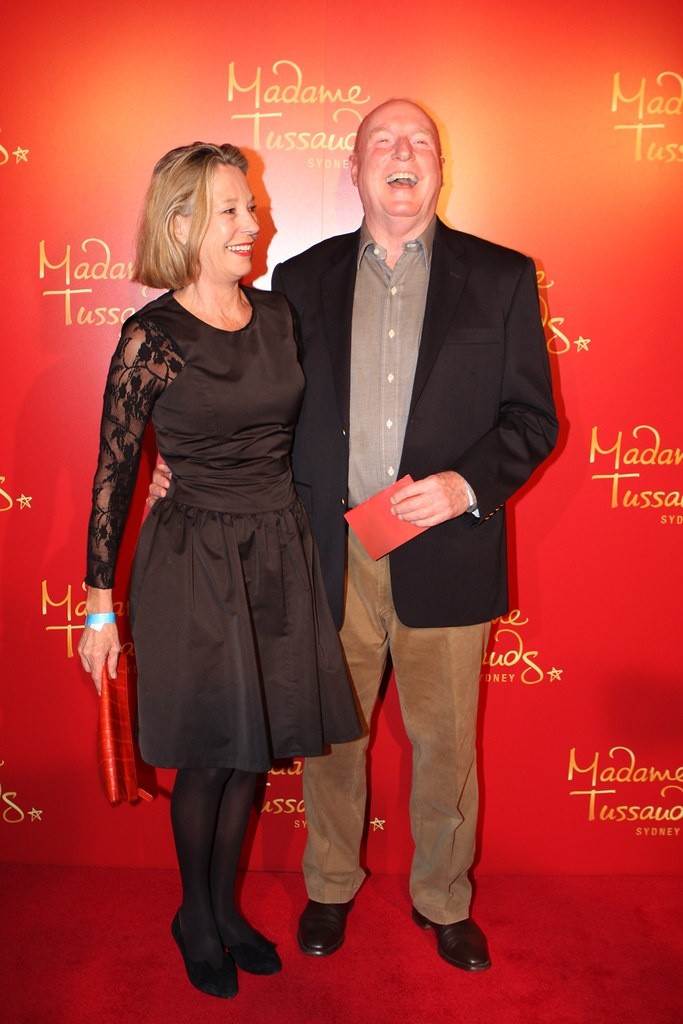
[171,906,283,999]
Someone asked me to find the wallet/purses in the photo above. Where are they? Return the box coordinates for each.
[94,650,159,809]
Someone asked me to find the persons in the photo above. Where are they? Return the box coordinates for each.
[145,98,560,971]
[78,140,365,1001]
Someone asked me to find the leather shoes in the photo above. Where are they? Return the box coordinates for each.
[297,897,356,957]
[411,904,492,972]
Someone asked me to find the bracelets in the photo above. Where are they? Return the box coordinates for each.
[85,612,116,632]
